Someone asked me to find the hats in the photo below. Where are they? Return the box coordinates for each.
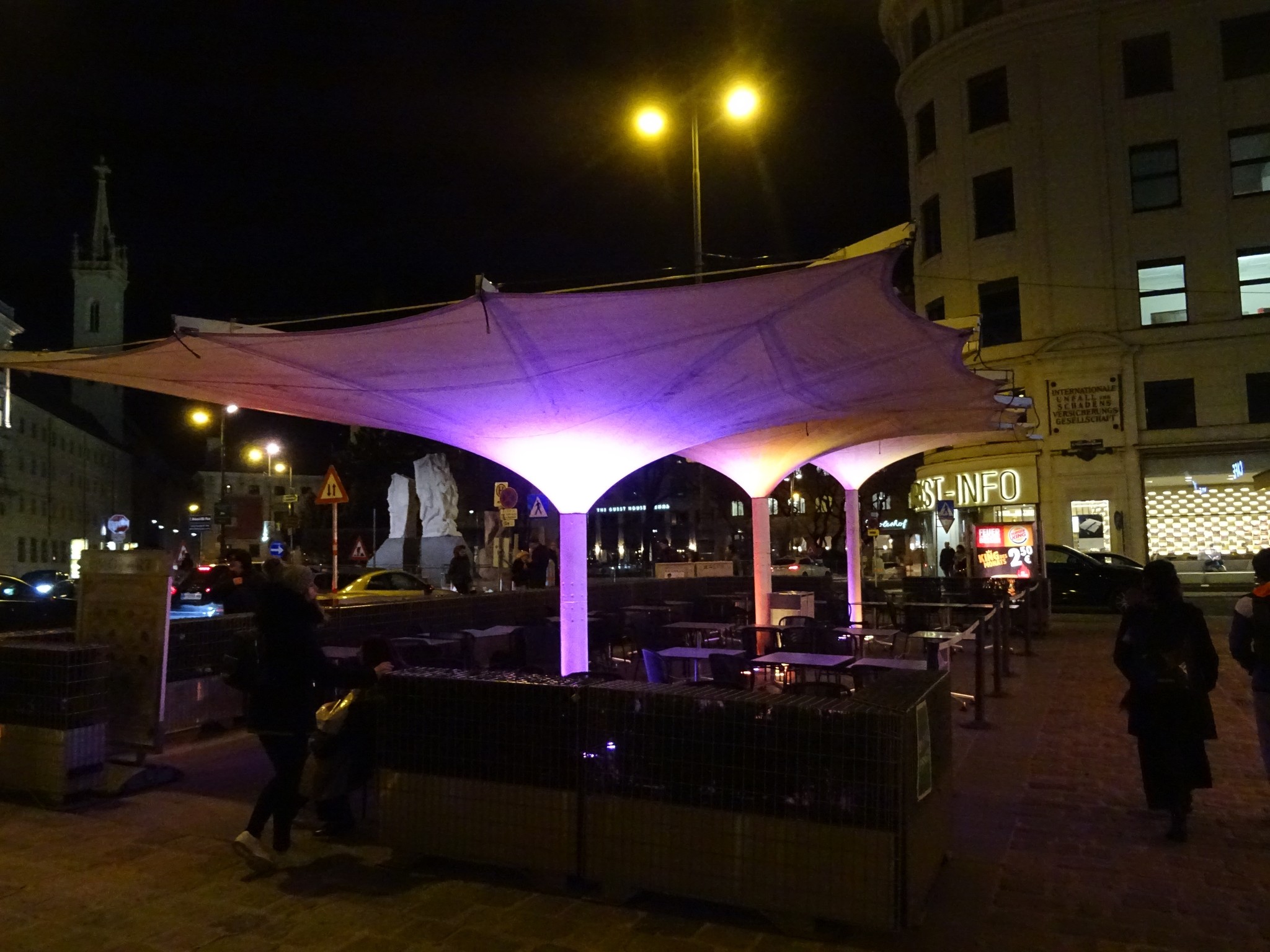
[264,557,313,596]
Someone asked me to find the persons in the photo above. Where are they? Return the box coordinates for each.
[181,553,193,570]
[1228,549,1270,813]
[511,538,560,588]
[952,545,966,575]
[203,550,284,613]
[1113,559,1218,842]
[940,541,955,576]
[679,549,699,563]
[655,540,679,562]
[723,545,741,576]
[231,563,393,871]
[446,545,473,594]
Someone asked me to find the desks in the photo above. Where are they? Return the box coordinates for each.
[621,605,674,633]
[844,657,949,679]
[907,629,976,660]
[967,603,1020,653]
[901,600,967,635]
[654,645,747,687]
[834,625,901,657]
[661,620,736,647]
[749,651,854,686]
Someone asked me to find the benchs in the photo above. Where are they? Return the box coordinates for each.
[1169,557,1258,589]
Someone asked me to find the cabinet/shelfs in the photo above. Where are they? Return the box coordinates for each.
[863,600,899,630]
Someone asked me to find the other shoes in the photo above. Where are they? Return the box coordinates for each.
[233,831,273,874]
[274,845,316,870]
[316,819,354,837]
[1169,789,1189,841]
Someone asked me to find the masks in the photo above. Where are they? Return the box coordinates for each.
[460,552,466,557]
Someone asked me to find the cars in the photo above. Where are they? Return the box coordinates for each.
[948,543,1146,615]
[170,560,459,621]
[0,570,80,633]
[771,556,832,577]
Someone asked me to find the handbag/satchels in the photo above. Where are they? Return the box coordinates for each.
[315,688,359,734]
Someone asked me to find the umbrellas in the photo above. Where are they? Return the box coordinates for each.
[677,395,1034,663]
[811,428,1047,638]
[0,220,1012,677]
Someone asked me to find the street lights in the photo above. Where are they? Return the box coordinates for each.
[250,443,280,540]
[628,72,761,285]
[190,404,238,558]
[275,464,295,553]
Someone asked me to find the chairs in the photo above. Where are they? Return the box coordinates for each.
[637,572,1000,696]
